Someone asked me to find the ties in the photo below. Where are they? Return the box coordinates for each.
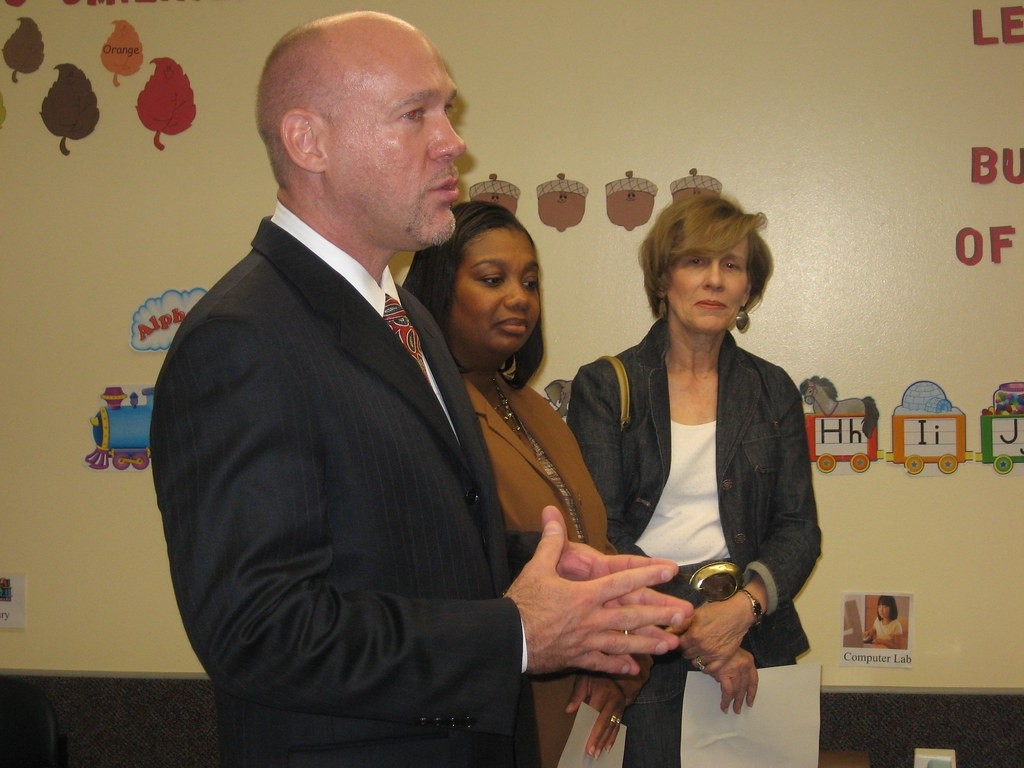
[383,295,423,378]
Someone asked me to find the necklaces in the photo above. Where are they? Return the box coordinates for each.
[493,379,520,431]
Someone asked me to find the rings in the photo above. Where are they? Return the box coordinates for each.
[617,719,620,723]
[697,657,705,670]
[625,630,627,634]
[611,716,616,722]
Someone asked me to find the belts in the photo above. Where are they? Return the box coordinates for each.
[678,559,744,599]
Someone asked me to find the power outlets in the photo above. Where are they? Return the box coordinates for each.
[914,748,956,768]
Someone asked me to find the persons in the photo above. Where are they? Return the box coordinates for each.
[149,11,693,768]
[864,596,902,649]
[567,195,822,768]
[402,201,656,768]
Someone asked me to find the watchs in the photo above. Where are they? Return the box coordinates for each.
[739,589,762,625]
